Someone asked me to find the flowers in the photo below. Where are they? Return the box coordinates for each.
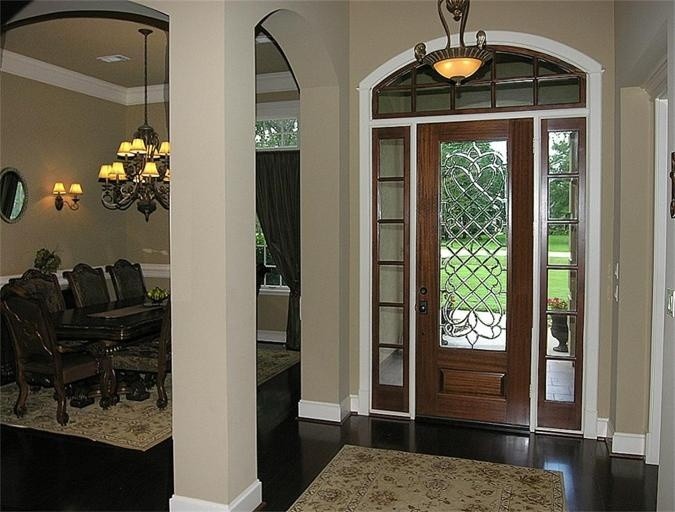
[546,297,570,312]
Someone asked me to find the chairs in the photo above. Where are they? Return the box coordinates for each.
[0,259,171,425]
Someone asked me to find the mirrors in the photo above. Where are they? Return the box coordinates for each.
[0,167,29,224]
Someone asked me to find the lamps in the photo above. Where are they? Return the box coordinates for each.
[52,183,83,210]
[414,0,495,88]
[99,26,170,221]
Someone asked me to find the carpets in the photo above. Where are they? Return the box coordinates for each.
[285,443,569,511]
[1,350,300,452]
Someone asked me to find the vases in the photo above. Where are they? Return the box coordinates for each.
[441,309,452,345]
[550,314,568,352]
